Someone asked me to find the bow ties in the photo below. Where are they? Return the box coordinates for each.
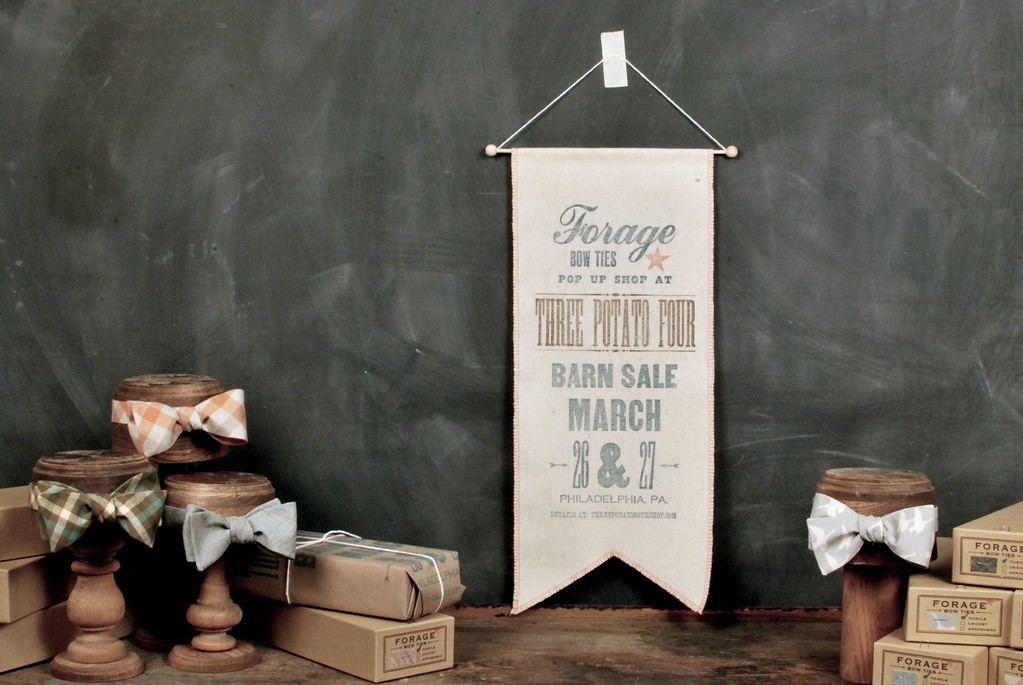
[165,498,297,571]
[806,492,939,575]
[30,473,165,552]
[110,387,247,457]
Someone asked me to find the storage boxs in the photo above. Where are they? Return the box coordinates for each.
[269,604,455,684]
[0,485,137,673]
[873,628,989,685]
[903,568,1013,646]
[951,501,1023,589]
[989,647,1023,685]
